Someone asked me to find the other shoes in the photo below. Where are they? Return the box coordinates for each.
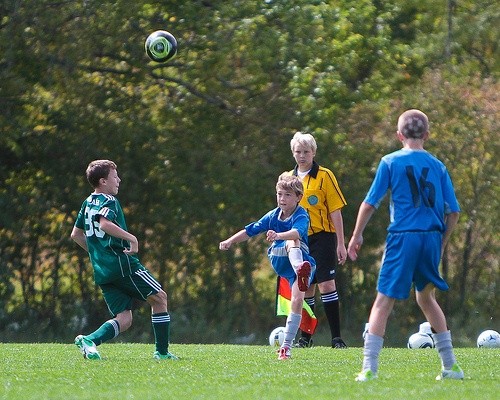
[435,365,463,381]
[292,338,313,348]
[355,370,378,381]
[331,337,347,349]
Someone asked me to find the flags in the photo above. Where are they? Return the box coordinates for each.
[277,277,317,334]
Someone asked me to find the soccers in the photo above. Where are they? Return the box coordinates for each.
[269,327,294,347]
[419,321,434,337]
[145,29,177,64]
[477,330,500,348]
[407,332,435,348]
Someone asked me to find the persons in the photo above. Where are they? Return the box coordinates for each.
[289,131,348,348]
[72,160,181,360]
[348,110,464,381]
[219,173,317,359]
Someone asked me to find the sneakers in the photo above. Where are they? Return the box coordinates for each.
[75,335,102,360]
[153,351,179,359]
[278,346,291,360]
[297,261,312,292]
